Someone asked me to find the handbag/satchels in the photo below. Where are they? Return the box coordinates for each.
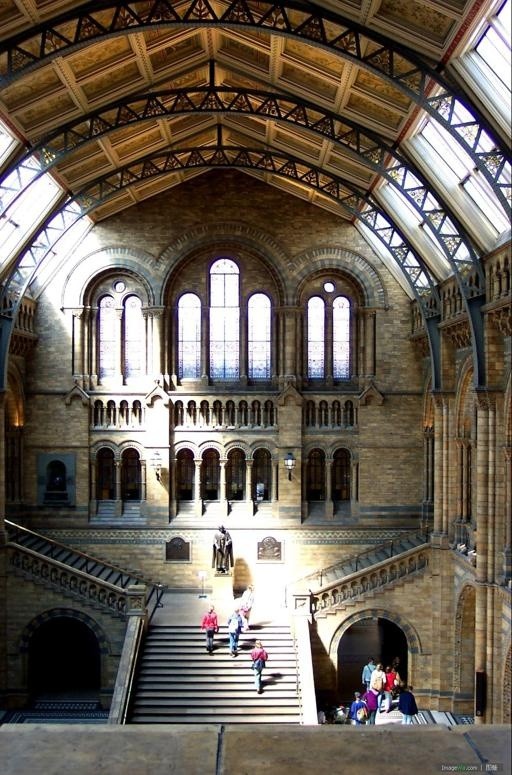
[207,627,215,637]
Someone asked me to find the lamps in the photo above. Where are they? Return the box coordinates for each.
[150,451,163,481]
[284,453,296,481]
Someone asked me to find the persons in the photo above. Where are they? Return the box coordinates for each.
[239,583,254,631]
[201,604,219,652]
[226,607,244,657]
[250,639,268,694]
[213,524,234,571]
[318,655,418,726]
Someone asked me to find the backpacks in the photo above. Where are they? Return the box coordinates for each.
[356,709,368,722]
[372,679,383,692]
[229,620,238,633]
[254,657,264,671]
